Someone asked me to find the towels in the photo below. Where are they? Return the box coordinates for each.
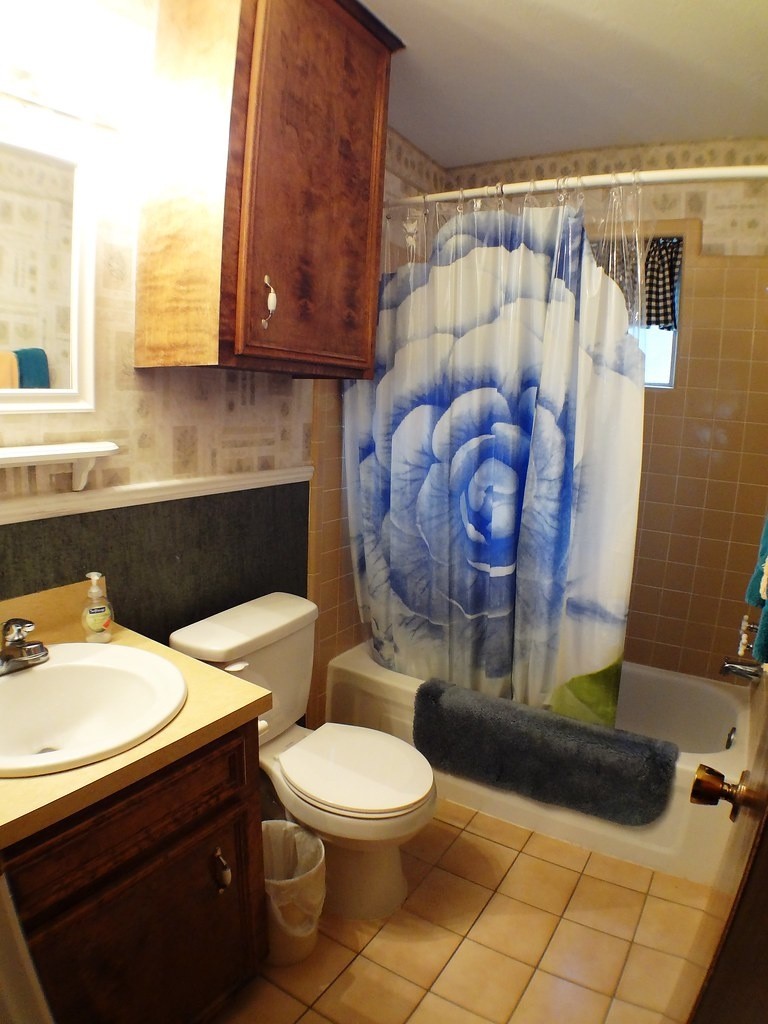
[0,348,51,388]
[414,678,678,825]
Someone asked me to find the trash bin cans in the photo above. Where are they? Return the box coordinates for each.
[258,817,328,968]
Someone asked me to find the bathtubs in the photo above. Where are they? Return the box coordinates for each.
[323,636,748,886]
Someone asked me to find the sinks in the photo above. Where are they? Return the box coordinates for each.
[0,643,188,778]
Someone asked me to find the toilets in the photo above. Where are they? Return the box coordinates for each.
[169,592,436,922]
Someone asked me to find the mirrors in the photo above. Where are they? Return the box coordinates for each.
[0,74,101,414]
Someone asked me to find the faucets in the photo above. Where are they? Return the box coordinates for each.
[719,656,762,685]
[0,619,50,676]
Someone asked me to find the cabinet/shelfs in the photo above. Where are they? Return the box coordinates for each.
[133,0,405,382]
[0,717,271,1024]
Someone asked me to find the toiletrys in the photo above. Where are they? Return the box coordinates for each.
[79,572,114,642]
